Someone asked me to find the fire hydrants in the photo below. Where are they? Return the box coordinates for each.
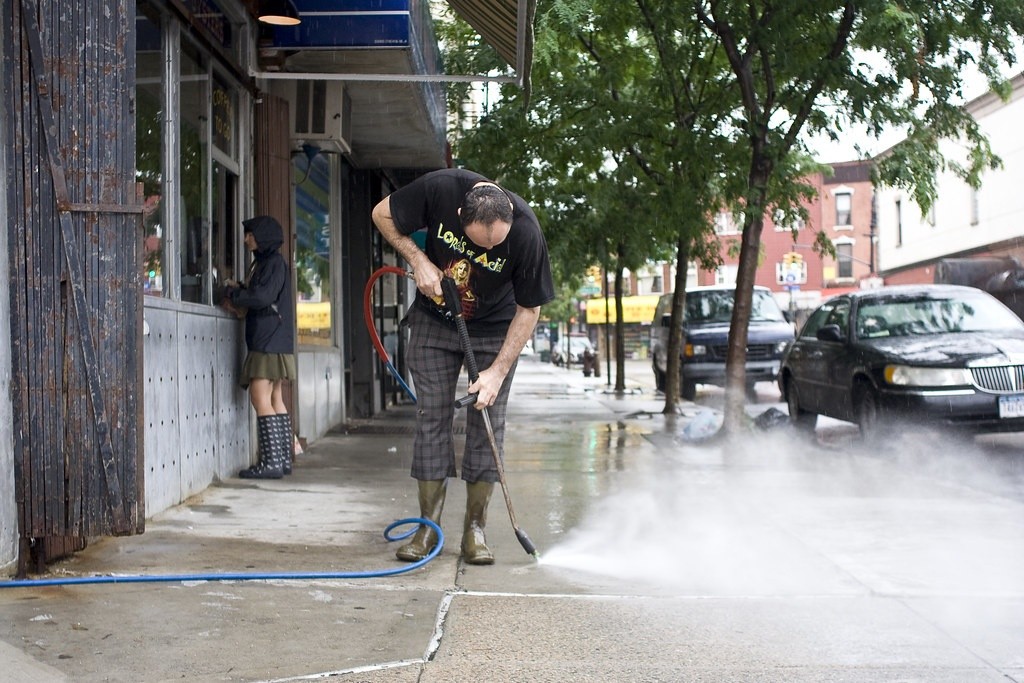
[581,345,594,377]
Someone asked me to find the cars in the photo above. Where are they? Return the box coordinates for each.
[552,332,595,368]
[778,282,1024,444]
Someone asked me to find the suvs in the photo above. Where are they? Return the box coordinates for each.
[650,280,797,402]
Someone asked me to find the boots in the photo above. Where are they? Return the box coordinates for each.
[249,413,292,475]
[238,416,284,479]
[396,478,449,560]
[460,480,495,564]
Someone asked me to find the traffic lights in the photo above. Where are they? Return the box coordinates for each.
[782,251,803,271]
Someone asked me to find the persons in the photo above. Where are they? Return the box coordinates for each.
[370,167,556,566]
[207,215,298,479]
